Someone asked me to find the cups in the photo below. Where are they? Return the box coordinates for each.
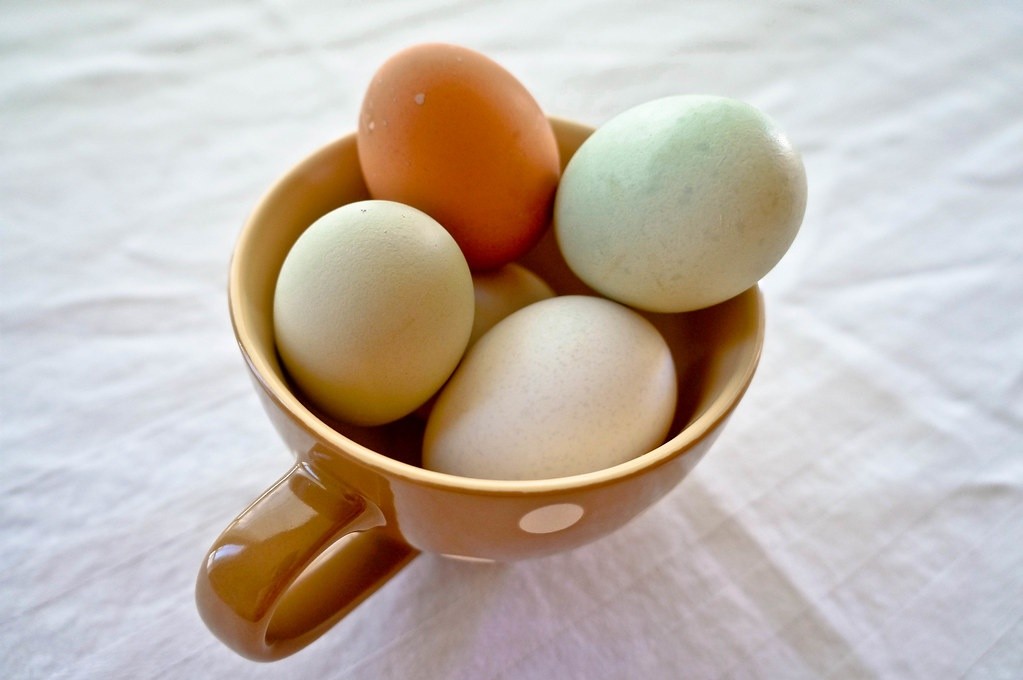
[195,115,767,664]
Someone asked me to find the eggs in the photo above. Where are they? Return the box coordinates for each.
[272,42,809,481]
